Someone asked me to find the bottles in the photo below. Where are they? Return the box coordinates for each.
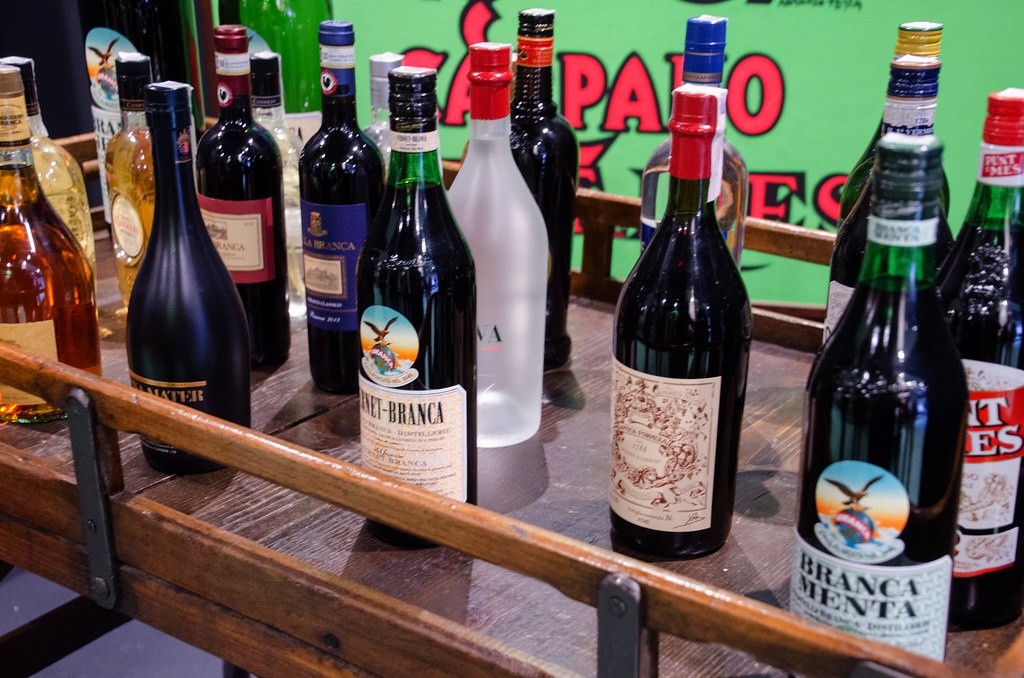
[609,13,754,563]
[1,55,103,426]
[793,19,1023,662]
[297,7,579,548]
[106,25,291,474]
